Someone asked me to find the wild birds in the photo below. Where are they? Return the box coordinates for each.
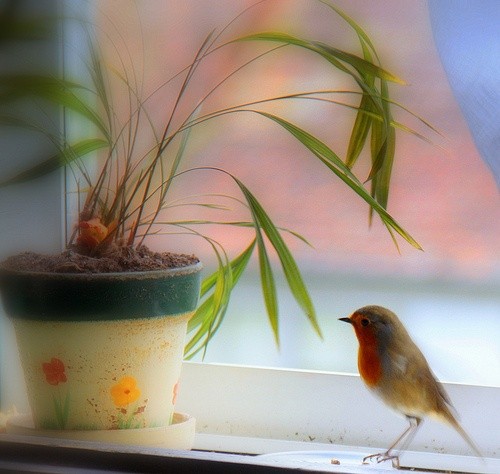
[337,306,485,472]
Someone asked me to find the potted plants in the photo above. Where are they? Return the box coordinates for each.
[2,0,448,453]
